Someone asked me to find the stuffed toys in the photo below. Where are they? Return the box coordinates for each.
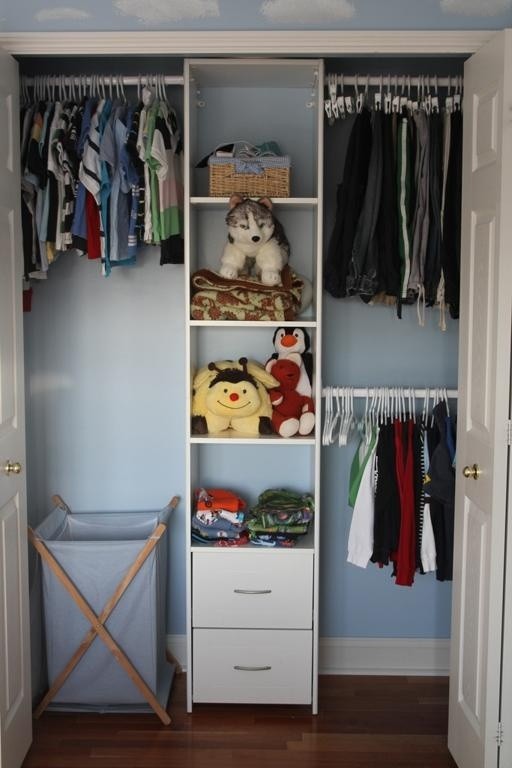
[190,357,282,436]
[215,190,290,287]
[265,358,316,439]
[265,327,313,400]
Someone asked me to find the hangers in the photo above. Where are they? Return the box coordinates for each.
[321,384,458,450]
[324,71,462,122]
[17,73,179,125]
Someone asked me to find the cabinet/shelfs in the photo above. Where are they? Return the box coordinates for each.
[179,52,326,715]
